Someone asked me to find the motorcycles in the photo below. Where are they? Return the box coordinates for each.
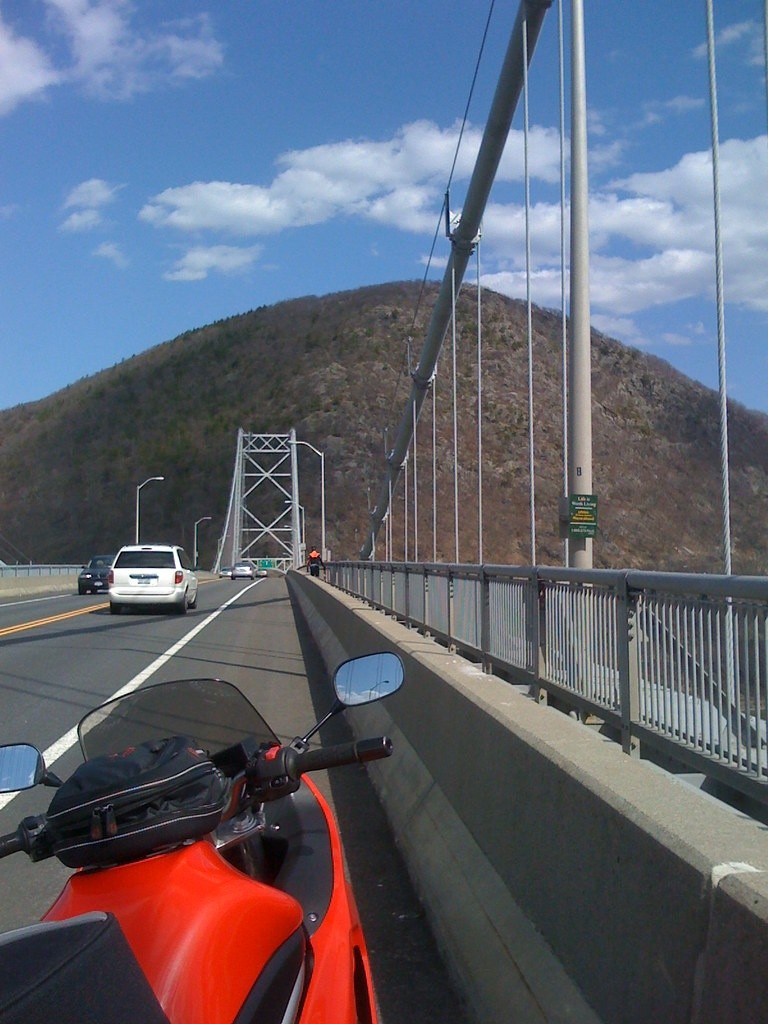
[0,652,405,1024]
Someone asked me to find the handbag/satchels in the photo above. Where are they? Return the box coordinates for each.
[43,734,226,868]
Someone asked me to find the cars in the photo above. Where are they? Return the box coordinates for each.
[107,544,198,615]
[219,567,233,578]
[256,568,268,577]
[78,555,116,595]
[231,562,257,580]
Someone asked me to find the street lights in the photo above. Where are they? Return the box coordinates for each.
[369,680,389,700]
[288,440,326,562]
[285,501,305,564]
[194,517,212,567]
[136,476,164,544]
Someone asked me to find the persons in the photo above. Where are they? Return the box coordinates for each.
[307,546,326,577]
